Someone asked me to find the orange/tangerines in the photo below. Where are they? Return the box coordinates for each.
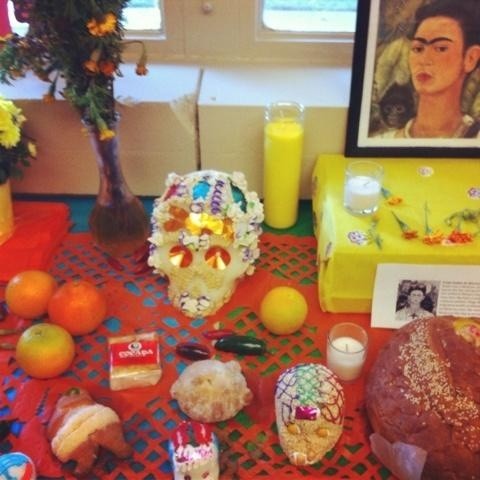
[49,282,108,337]
[4,270,57,320]
[14,323,75,378]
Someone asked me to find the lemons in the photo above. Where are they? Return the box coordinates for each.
[260,286,309,335]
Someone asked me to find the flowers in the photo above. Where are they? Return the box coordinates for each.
[0,99,38,183]
[1,1,148,141]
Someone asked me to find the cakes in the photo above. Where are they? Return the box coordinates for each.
[273,362,345,467]
[165,422,223,480]
[169,358,253,427]
[146,171,268,318]
[363,316,479,479]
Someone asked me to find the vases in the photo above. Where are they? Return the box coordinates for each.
[0,180,16,245]
[86,110,151,240]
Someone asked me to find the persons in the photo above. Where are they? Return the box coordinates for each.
[396,286,435,321]
[365,0,480,139]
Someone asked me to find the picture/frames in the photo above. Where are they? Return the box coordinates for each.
[346,1,479,158]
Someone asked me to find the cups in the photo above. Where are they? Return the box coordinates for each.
[344,161,383,216]
[326,322,369,381]
[262,101,305,229]
